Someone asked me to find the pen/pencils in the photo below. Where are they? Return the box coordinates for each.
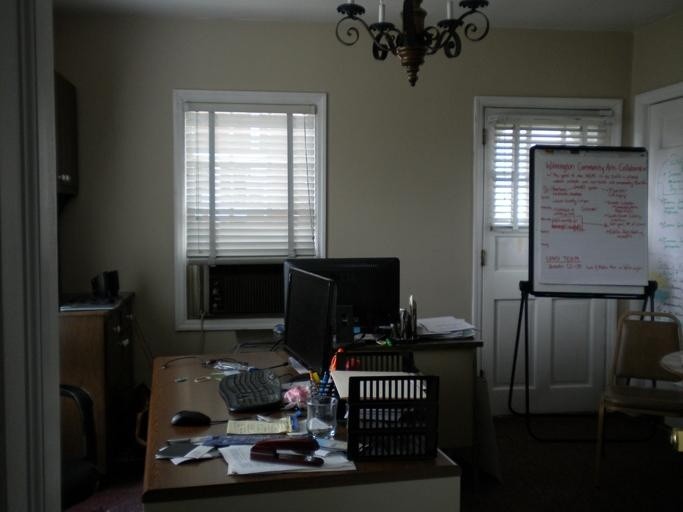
[308,369,332,398]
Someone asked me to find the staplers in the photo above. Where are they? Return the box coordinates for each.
[249,438,323,466]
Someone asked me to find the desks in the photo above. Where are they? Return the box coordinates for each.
[139,328,484,511]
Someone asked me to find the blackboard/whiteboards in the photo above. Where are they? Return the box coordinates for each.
[527,145,649,298]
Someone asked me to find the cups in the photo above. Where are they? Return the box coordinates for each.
[305,396,339,439]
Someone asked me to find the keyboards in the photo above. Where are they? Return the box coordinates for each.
[217,370,281,414]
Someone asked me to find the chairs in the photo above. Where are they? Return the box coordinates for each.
[594,309,681,483]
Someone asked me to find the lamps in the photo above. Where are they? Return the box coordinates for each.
[332,0,493,90]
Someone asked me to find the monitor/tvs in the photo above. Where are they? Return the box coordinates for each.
[286,257,400,350]
[283,266,338,383]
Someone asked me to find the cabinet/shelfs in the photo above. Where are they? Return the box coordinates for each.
[53,69,80,197]
[58,291,134,472]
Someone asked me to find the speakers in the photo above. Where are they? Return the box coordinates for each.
[91,270,119,305]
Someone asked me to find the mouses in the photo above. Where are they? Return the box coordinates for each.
[171,411,210,426]
